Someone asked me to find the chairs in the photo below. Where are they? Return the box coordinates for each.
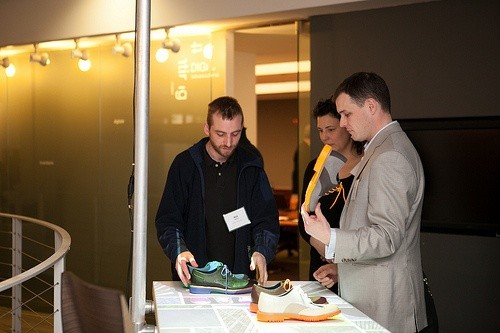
[61,271,133,333]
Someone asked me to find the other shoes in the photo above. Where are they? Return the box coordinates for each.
[257,286,341,323]
[180,261,250,289]
[190,266,258,294]
[250,279,329,313]
[303,144,348,213]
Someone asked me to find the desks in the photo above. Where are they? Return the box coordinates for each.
[152,279,390,333]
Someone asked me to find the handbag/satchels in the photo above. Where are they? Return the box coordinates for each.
[422,273,439,333]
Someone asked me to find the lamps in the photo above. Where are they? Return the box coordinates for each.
[29,43,49,65]
[0,57,10,68]
[163,25,181,53]
[71,39,88,61]
[112,34,134,57]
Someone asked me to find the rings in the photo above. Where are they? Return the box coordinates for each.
[305,220,309,225]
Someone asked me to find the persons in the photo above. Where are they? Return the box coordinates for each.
[155,96,280,287]
[301,71,428,333]
[290,148,299,210]
[298,97,367,296]
